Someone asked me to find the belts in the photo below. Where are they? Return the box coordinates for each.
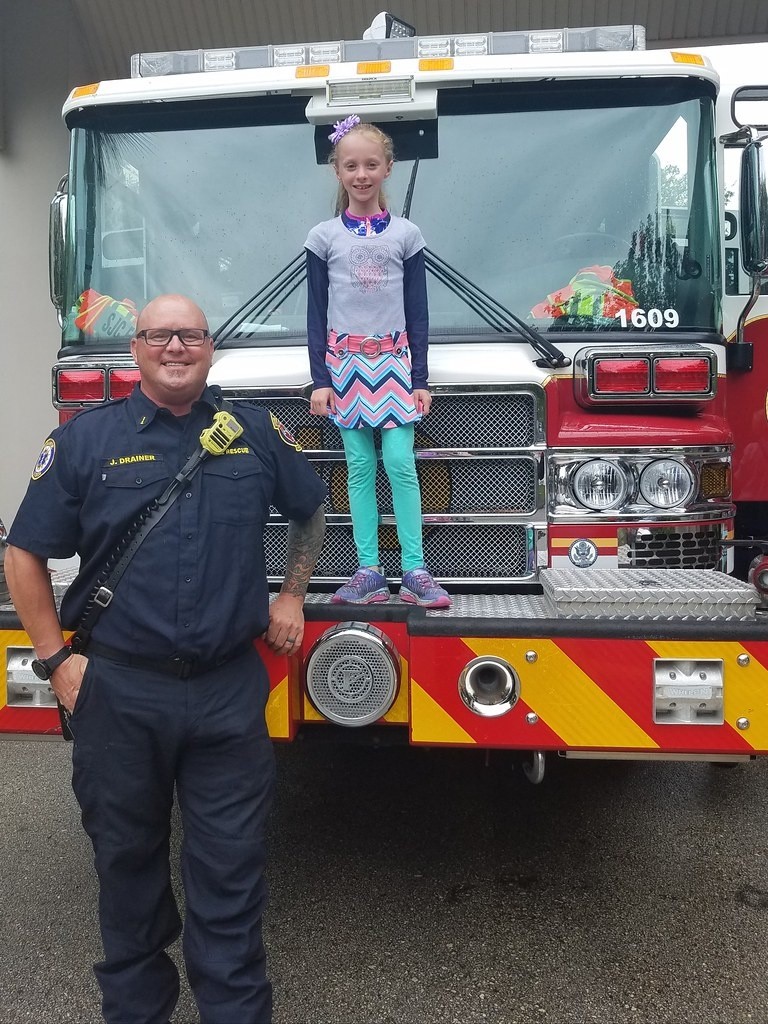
[86,641,248,681]
[327,331,408,359]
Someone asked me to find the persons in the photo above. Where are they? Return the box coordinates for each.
[304,113,452,609]
[3,291,330,1024]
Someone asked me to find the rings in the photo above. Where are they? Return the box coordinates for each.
[286,637,295,643]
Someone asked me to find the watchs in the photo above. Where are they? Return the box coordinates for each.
[31,646,71,681]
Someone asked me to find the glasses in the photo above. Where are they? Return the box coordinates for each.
[136,328,210,346]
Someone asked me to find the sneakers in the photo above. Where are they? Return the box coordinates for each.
[399,563,454,608]
[331,565,390,605]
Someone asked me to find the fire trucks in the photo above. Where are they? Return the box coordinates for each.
[0,9,768,788]
